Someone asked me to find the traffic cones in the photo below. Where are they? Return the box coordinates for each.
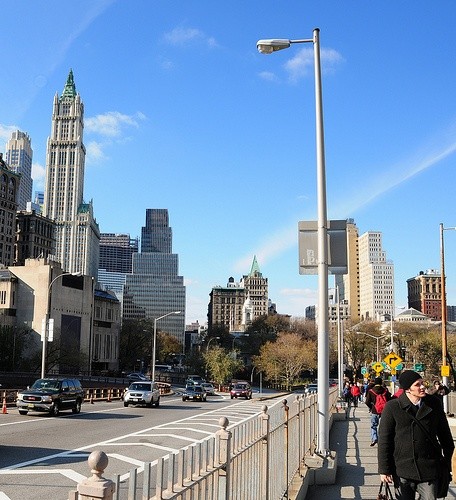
[0,399,9,414]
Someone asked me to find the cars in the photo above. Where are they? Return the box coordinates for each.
[185,375,216,395]
[230,382,252,400]
[182,386,208,402]
[127,372,149,381]
[302,383,317,394]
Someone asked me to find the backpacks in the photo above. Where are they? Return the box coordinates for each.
[369,389,387,413]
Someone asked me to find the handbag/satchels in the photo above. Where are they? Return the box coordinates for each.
[434,455,452,498]
[377,482,394,500]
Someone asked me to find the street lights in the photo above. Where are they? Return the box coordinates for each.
[256,28,331,456]
[205,337,221,377]
[152,311,181,382]
[328,285,341,399]
[41,272,81,378]
[357,331,399,361]
[390,306,406,349]
[232,334,249,351]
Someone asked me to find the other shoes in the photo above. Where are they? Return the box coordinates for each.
[370,439,378,446]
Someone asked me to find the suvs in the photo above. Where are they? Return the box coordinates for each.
[122,382,160,409]
[17,377,85,417]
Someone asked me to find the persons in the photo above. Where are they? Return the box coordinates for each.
[304,374,449,448]
[376,371,454,500]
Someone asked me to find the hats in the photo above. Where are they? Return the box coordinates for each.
[399,370,422,389]
